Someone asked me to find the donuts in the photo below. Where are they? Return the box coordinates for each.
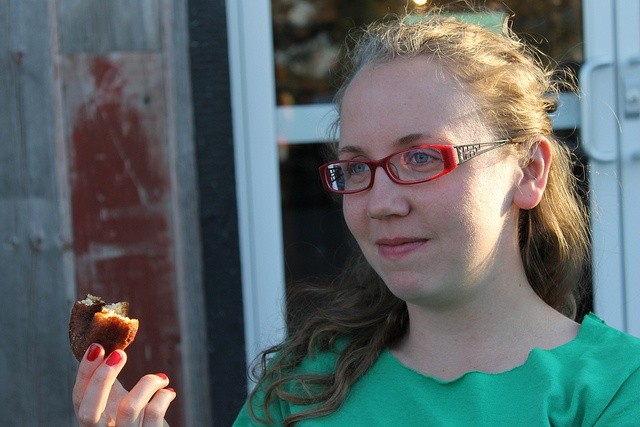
[68,296,139,363]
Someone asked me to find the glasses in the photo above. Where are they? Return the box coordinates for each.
[318,139,523,193]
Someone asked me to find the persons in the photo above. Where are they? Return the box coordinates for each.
[73,0,636,426]
